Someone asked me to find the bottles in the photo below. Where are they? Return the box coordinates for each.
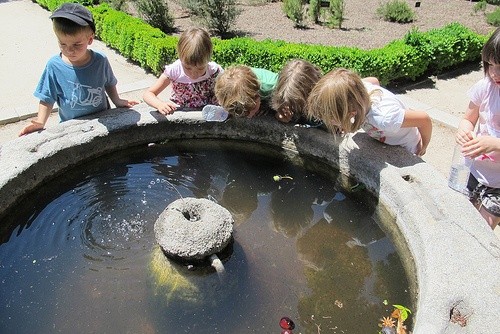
[447,130,475,191]
[201,105,228,122]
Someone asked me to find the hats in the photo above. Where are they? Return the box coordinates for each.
[49,3,96,33]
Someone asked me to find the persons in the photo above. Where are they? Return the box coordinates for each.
[307,68,433,156]
[270,58,323,123]
[455,25,500,230]
[214,64,279,117]
[18,3,139,137]
[142,27,225,115]
